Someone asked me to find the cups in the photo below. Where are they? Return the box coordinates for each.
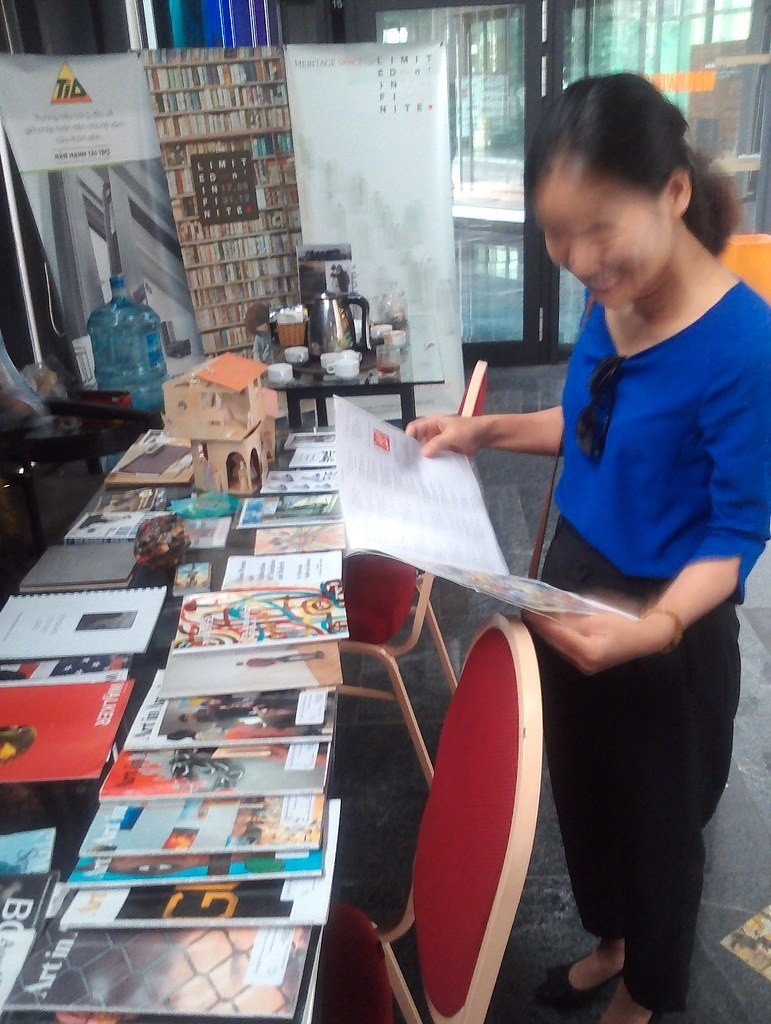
[320,350,363,380]
[369,323,406,379]
[285,346,309,365]
[268,363,293,386]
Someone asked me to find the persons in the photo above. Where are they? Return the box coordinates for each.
[404,73,771,1024]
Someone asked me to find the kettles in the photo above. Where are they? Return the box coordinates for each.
[307,290,372,360]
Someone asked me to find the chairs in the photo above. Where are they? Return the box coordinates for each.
[337,359,489,784]
[310,611,544,1024]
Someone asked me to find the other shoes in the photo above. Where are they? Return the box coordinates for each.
[532,951,633,1007]
[647,1010,662,1024]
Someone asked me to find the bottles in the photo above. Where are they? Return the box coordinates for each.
[86,272,170,409]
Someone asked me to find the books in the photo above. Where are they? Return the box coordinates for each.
[0,432,351,1023]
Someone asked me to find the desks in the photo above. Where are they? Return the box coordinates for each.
[261,313,445,430]
[0,433,347,1024]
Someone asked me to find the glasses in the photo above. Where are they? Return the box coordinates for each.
[576,352,622,461]
